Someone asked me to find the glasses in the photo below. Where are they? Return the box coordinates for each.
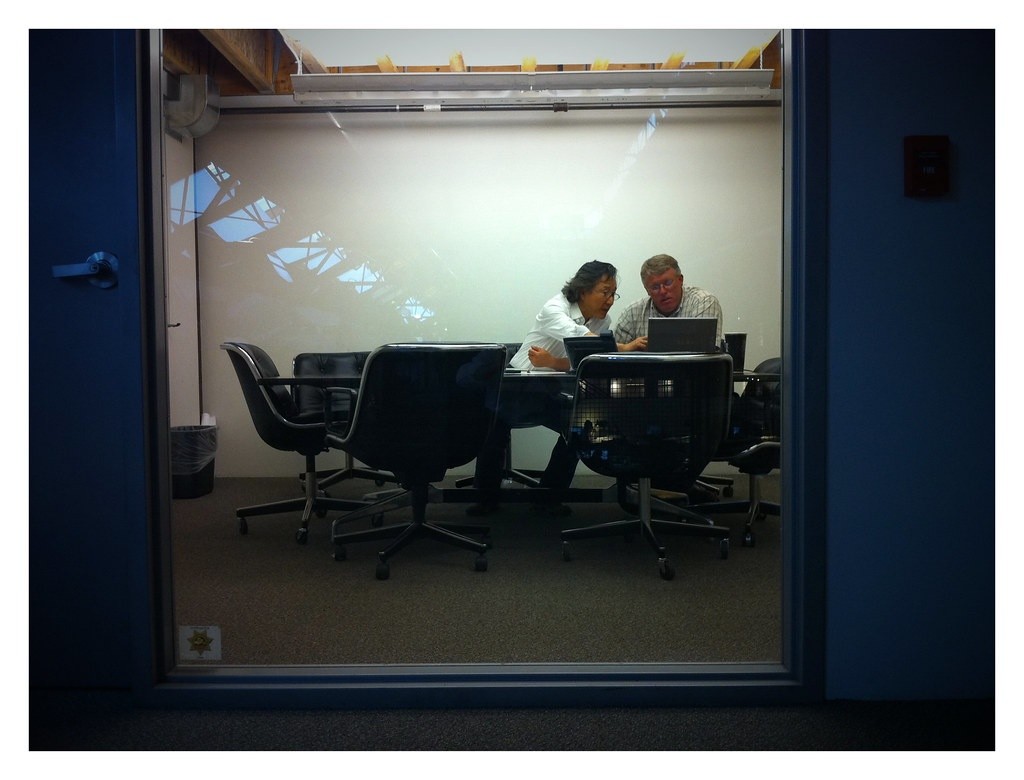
[595,288,620,301]
[647,276,680,293]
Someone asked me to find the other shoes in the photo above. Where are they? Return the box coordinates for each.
[466,502,499,515]
[529,504,571,515]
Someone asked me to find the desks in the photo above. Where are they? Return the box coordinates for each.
[256,370,780,543]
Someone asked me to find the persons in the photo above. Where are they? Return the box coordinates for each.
[466,261,647,517]
[613,254,723,503]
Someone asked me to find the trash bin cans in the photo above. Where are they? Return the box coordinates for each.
[169,425,217,500]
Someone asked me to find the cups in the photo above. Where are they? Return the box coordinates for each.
[724,332,747,373]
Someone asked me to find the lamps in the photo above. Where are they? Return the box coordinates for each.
[289,45,775,107]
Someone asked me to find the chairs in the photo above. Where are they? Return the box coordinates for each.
[455,342,546,489]
[683,357,780,549]
[218,341,385,546]
[323,342,508,581]
[558,352,733,582]
[291,352,401,498]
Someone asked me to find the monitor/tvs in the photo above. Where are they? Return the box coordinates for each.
[647,318,717,352]
[563,336,618,369]
